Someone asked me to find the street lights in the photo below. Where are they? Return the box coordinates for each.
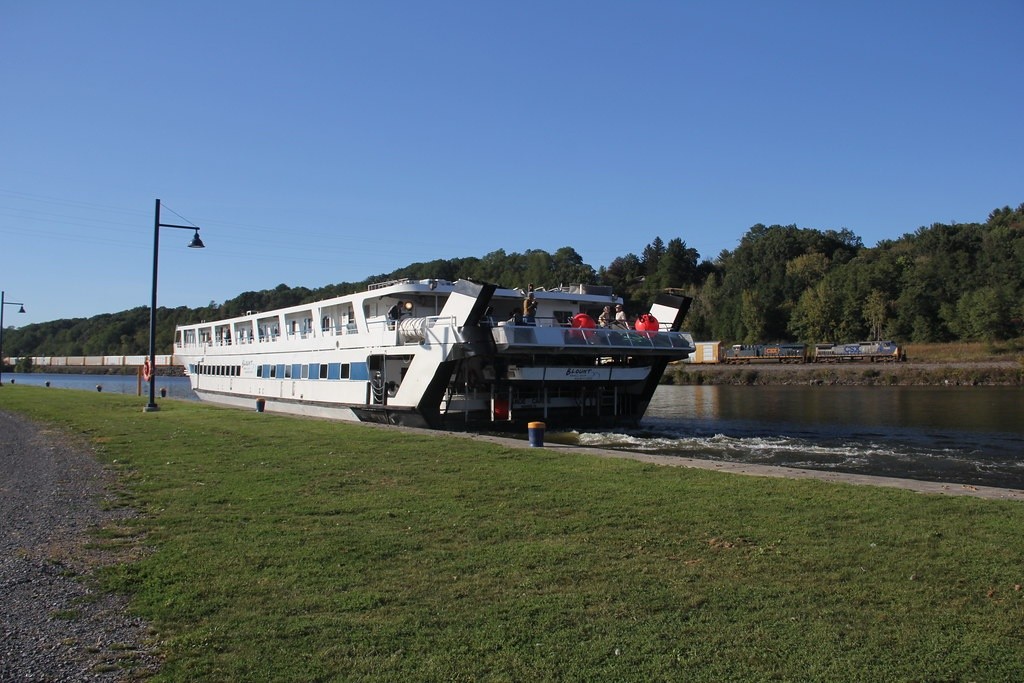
[0,289,26,387]
[143,196,205,413]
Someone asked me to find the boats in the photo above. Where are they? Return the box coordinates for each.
[169,276,700,434]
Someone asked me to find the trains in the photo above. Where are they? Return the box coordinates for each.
[671,340,906,365]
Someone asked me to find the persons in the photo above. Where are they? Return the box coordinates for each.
[524,292,536,326]
[224,329,231,345]
[615,305,627,325]
[599,306,610,328]
[387,300,404,330]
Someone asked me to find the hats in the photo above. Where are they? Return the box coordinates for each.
[615,305,622,308]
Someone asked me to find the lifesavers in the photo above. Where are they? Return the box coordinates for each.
[143,360,152,382]
